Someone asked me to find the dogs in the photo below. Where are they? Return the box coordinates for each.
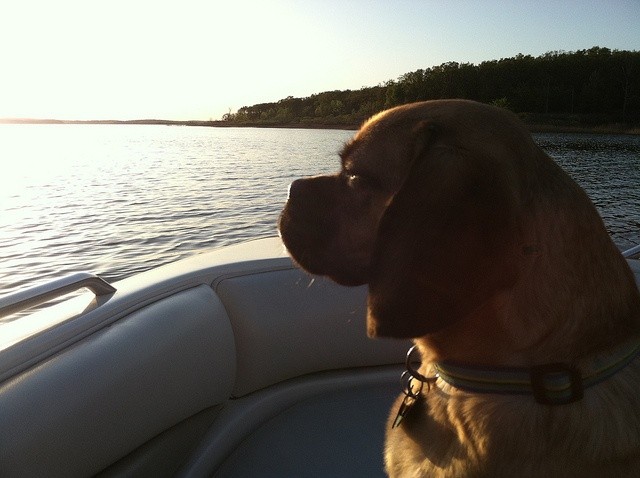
[277,98,640,478]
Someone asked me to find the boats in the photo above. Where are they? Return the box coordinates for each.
[0,237,640,478]
